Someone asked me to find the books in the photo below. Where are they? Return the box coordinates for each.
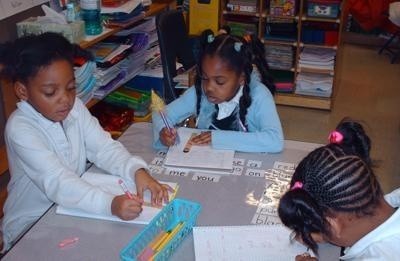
[192,226,316,261]
[162,127,235,173]
[266,45,335,98]
[74,0,162,132]
[56,172,180,224]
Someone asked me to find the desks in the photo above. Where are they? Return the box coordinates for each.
[0,123,344,261]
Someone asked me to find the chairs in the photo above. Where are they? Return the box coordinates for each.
[155,62,273,129]
[376,14,399,65]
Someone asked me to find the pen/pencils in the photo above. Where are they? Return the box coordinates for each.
[119,180,134,200]
[304,246,310,253]
[136,221,184,261]
[151,90,177,147]
[60,236,81,248]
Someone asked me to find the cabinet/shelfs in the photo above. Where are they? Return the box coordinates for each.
[0,0,173,261]
[219,0,345,112]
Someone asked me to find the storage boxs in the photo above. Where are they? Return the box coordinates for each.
[188,0,219,37]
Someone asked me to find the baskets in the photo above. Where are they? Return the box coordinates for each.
[119,198,201,261]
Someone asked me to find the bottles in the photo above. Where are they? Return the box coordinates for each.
[65,3,77,24]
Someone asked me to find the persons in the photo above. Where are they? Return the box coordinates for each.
[0,32,176,254]
[152,25,284,153]
[277,117,400,261]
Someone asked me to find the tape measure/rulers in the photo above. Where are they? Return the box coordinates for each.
[183,132,197,153]
[141,202,164,209]
